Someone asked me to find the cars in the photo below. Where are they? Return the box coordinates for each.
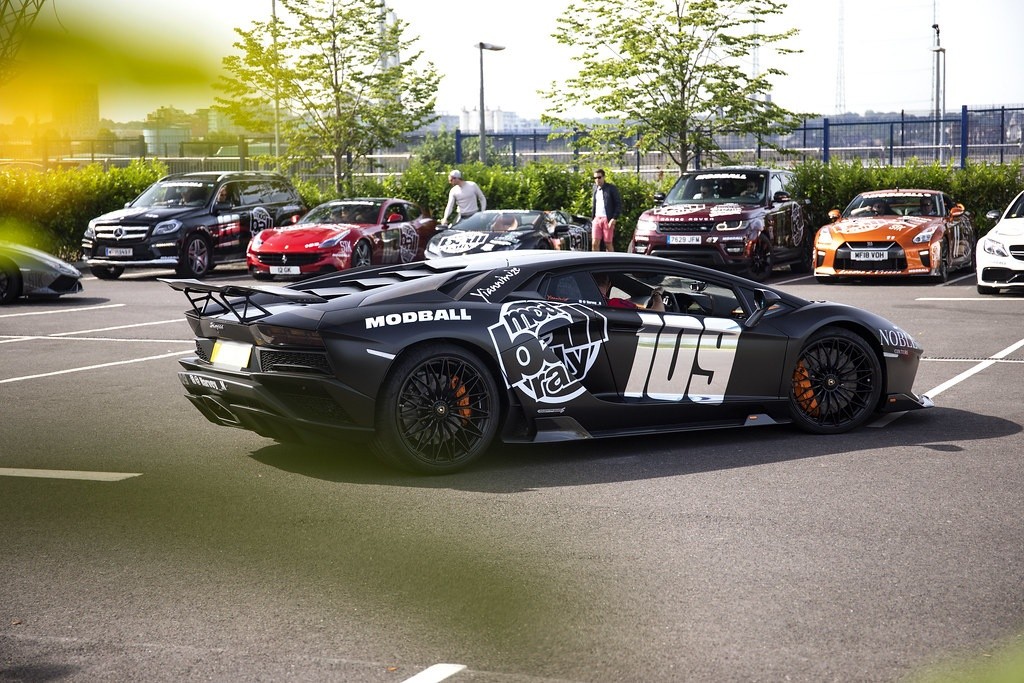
[811,189,974,285]
[977,190,1024,295]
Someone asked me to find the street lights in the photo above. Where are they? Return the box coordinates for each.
[474,42,505,167]
[932,46,946,169]
[932,24,941,165]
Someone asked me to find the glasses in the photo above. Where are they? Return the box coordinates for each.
[747,186,756,189]
[593,175,604,179]
[449,177,455,181]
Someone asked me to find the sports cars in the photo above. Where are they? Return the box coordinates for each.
[156,249,933,477]
[247,198,435,281]
[424,210,592,259]
[0,241,83,307]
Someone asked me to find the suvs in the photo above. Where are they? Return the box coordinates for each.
[628,167,811,283]
[82,170,312,279]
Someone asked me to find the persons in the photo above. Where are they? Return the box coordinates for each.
[739,180,762,199]
[152,187,192,208]
[912,197,937,216]
[592,272,665,312]
[326,206,400,225]
[218,186,229,204]
[700,183,715,200]
[441,169,487,225]
[591,169,621,252]
[851,200,889,215]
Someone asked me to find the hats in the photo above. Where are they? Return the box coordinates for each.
[450,170,461,178]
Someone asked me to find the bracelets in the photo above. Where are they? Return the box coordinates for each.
[651,292,663,299]
